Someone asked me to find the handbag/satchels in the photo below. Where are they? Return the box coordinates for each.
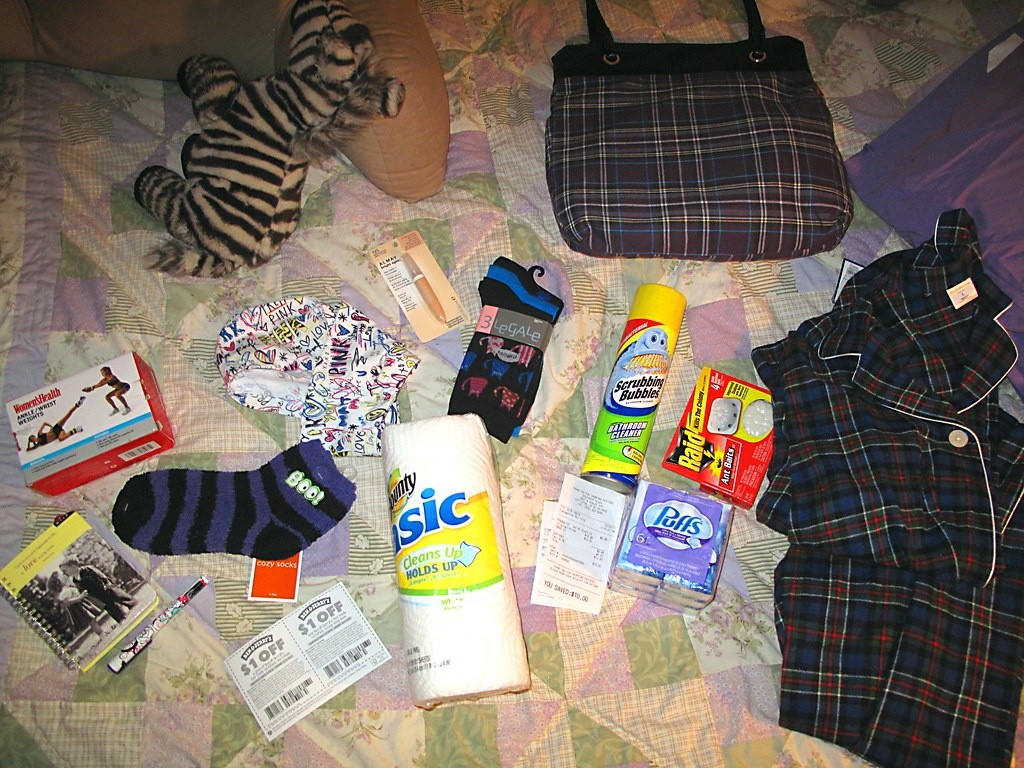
[545,0,855,261]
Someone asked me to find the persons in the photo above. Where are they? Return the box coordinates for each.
[59,555,138,624]
[83,367,130,416]
[47,572,107,640]
[26,396,86,451]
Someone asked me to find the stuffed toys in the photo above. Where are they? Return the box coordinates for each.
[133,0,406,279]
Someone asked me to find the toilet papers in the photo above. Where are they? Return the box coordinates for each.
[377,413,535,712]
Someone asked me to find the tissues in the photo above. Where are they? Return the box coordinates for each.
[606,478,736,619]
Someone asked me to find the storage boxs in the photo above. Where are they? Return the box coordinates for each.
[662,365,773,509]
[5,353,177,497]
